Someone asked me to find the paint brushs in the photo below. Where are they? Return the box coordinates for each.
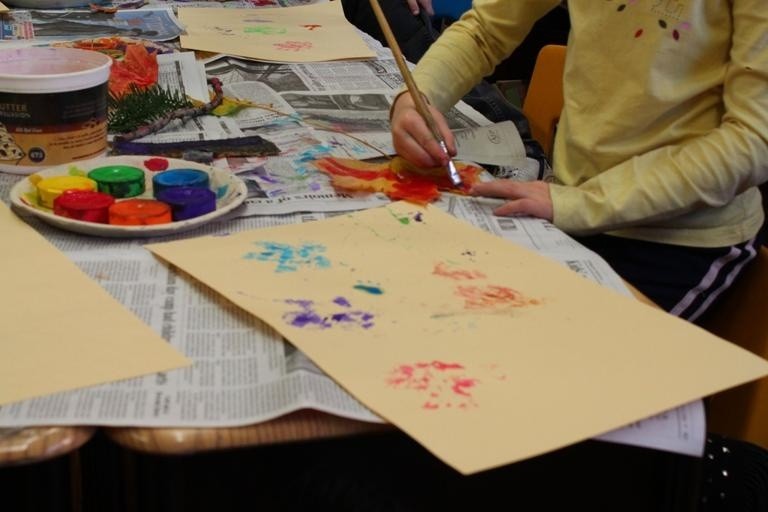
[371,0,465,190]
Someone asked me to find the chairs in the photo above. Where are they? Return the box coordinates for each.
[522,44,570,163]
[694,243,768,511]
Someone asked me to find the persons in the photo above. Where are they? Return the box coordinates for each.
[343,0,549,188]
[389,1,768,321]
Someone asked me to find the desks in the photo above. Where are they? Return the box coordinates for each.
[102,270,661,512]
[0,425,103,512]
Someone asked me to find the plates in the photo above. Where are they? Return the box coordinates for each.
[9,155,248,238]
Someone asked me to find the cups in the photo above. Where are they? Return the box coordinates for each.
[0,44,113,177]
